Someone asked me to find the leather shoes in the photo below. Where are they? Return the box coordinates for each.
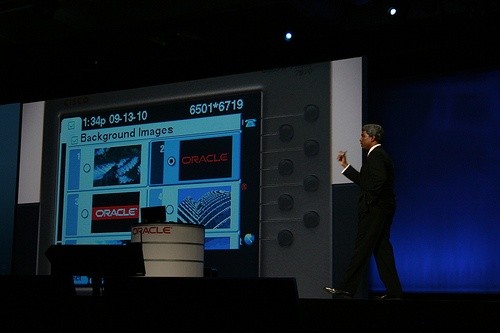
[324,285,354,298]
[380,292,402,300]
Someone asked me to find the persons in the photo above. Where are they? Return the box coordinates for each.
[323,122,408,301]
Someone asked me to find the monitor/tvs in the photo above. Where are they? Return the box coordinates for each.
[140,206,167,223]
